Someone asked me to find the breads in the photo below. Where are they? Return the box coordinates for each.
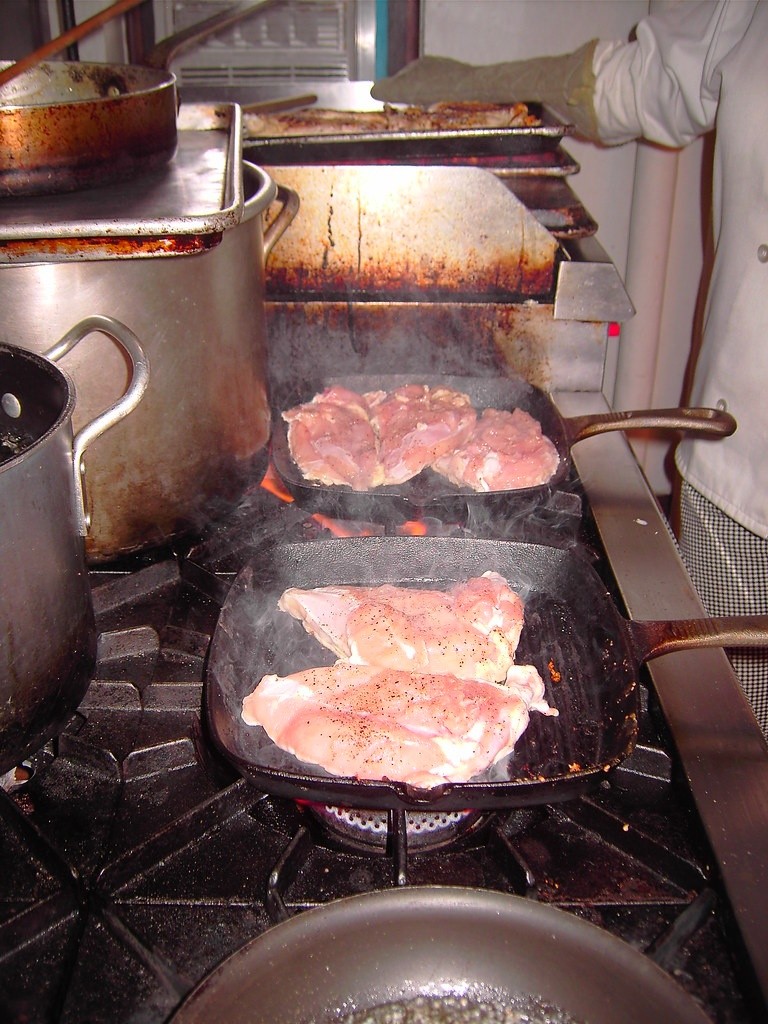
[242,94,534,145]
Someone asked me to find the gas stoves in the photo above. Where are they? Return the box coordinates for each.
[0,389,768,1024]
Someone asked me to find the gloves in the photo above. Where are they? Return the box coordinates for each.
[370,38,600,141]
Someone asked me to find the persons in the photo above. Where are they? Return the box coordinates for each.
[371,1,767,739]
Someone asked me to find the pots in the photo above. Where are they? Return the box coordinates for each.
[0,314,151,776]
[166,883,713,1024]
[0,0,276,198]
[272,375,737,497]
[204,536,768,811]
[0,159,302,567]
